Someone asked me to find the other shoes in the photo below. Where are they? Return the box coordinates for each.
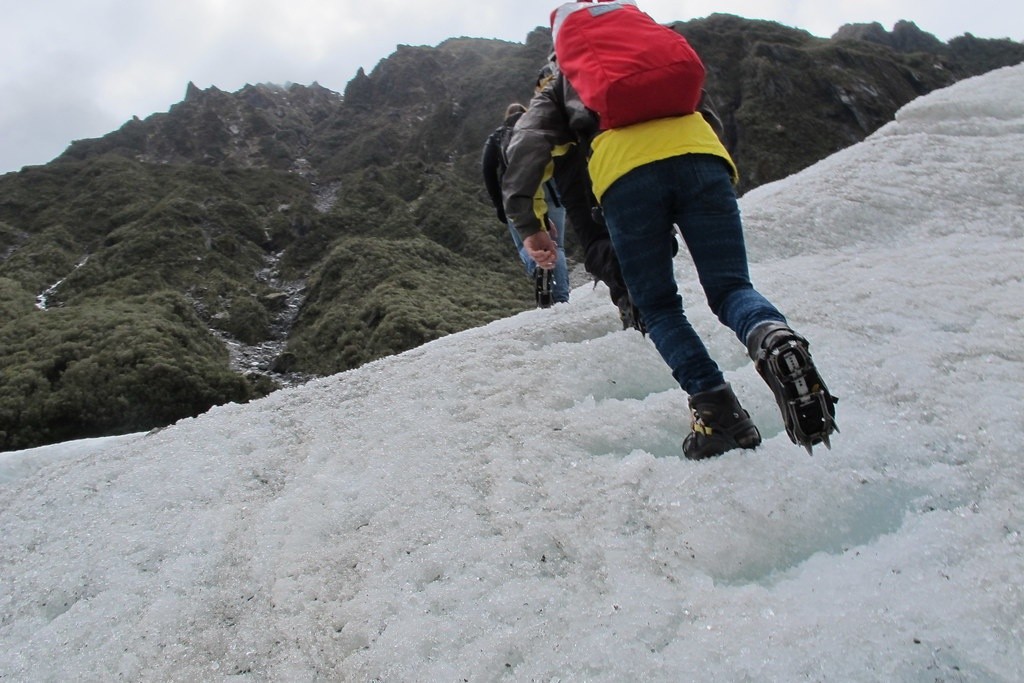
[535,266,557,309]
[617,295,636,330]
[672,234,678,257]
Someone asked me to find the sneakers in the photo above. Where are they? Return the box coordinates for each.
[682,382,761,461]
[745,320,840,457]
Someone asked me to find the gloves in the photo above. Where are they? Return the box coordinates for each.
[496,199,508,224]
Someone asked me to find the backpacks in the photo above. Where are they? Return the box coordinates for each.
[550,0,706,130]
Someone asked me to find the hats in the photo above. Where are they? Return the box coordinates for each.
[504,103,527,122]
[547,46,555,62]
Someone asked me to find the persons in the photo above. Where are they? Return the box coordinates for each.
[481,45,842,461]
